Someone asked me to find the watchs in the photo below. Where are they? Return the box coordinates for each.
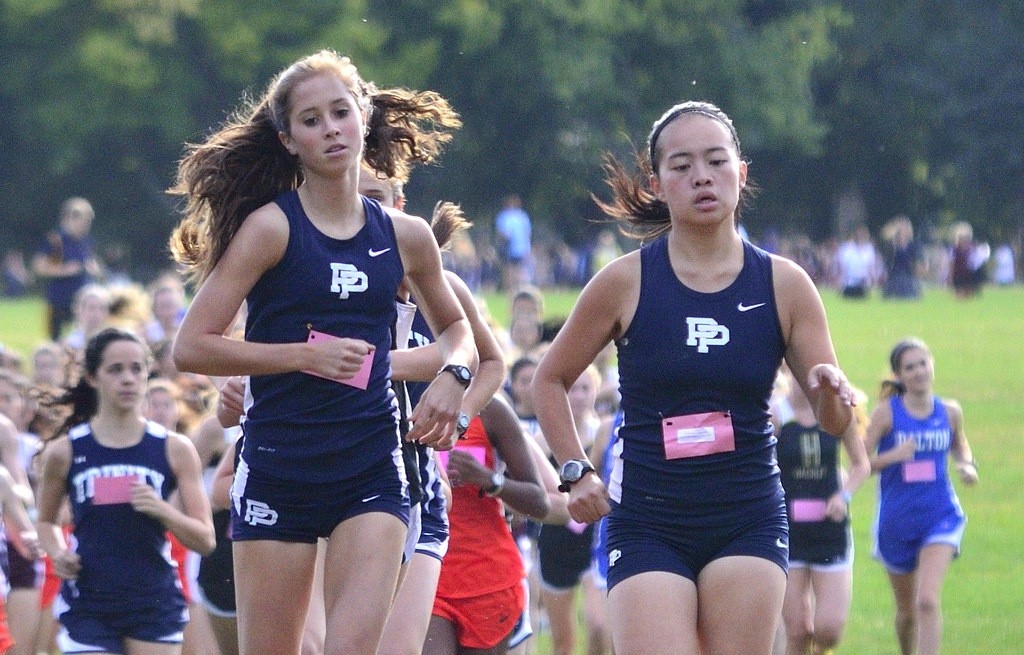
[557,459,595,493]
[839,490,852,502]
[456,410,470,439]
[437,364,471,390]
[477,471,505,498]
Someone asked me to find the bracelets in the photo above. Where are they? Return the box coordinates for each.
[485,487,503,497]
[966,461,978,471]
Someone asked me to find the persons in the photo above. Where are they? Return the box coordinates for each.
[0,51,1024,655]
